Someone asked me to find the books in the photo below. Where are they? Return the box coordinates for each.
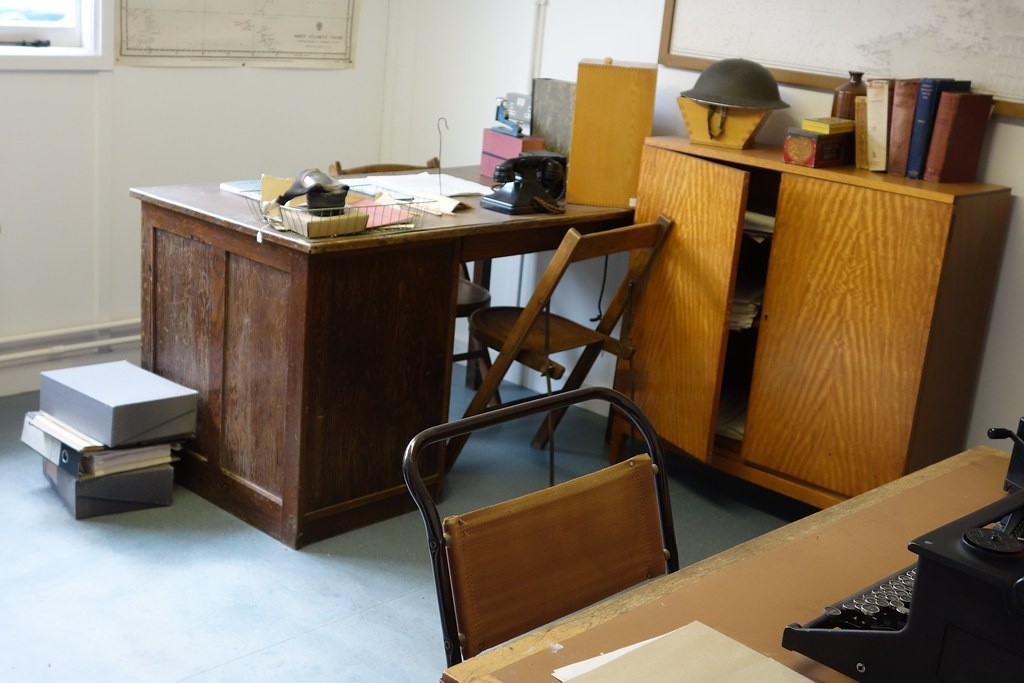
[802,77,994,184]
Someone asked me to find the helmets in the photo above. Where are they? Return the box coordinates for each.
[681,58,790,110]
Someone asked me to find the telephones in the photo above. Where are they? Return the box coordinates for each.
[480,154,569,215]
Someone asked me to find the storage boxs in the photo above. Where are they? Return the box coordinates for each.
[40,359,198,447]
[39,459,198,521]
[565,58,657,210]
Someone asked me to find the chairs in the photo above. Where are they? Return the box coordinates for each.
[331,156,673,474]
[404,387,681,666]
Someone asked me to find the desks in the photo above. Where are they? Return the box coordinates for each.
[127,163,635,550]
[439,444,1011,683]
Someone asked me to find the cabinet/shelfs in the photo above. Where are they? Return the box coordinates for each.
[610,136,1012,510]
